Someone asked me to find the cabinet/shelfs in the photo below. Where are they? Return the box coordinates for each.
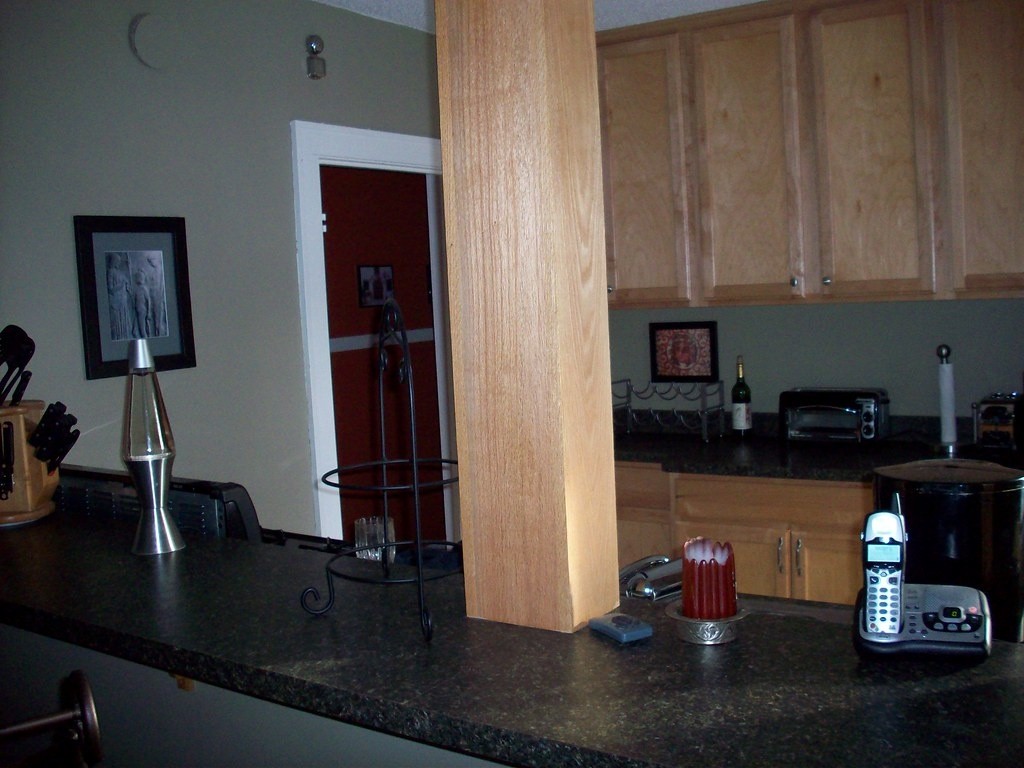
[593,0,1024,308]
[668,470,873,606]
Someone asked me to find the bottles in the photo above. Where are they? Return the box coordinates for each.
[732,355,751,440]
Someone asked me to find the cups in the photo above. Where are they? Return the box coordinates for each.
[355,516,396,561]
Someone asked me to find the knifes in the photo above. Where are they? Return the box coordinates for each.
[9,370,80,475]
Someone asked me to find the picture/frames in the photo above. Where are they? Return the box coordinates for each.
[356,264,395,308]
[649,321,720,383]
[73,215,197,380]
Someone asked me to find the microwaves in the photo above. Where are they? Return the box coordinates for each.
[779,385,891,447]
